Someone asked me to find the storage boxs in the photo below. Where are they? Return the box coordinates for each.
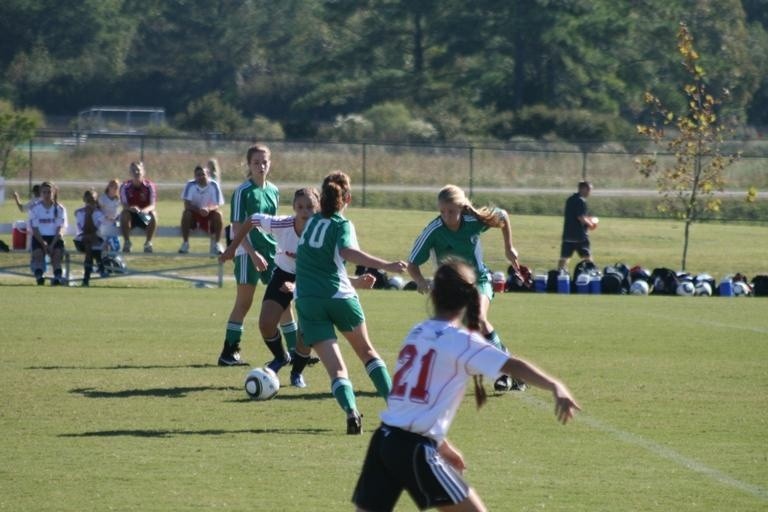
[477,258,748,298]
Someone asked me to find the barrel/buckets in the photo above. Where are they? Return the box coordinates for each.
[10,220,27,249]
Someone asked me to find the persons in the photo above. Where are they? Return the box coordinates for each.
[408,184,533,392]
[119,161,157,252]
[30,181,67,287]
[74,189,108,287]
[349,255,583,512]
[557,179,598,270]
[206,160,219,182]
[296,171,408,434]
[99,178,121,252]
[13,184,40,253]
[217,143,319,368]
[177,165,224,255]
[218,186,377,375]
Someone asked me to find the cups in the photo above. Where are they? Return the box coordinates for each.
[591,218,599,227]
[493,280,504,292]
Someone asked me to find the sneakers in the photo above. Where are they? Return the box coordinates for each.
[216,348,250,366]
[493,371,510,390]
[343,409,366,436]
[266,352,291,373]
[36,271,45,285]
[123,238,133,252]
[289,368,307,388]
[52,271,64,285]
[289,352,321,364]
[178,239,191,253]
[144,241,153,253]
[209,242,225,255]
[511,376,530,392]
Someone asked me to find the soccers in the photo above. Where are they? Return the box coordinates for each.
[244,368,279,401]
[630,281,648,296]
[732,283,749,296]
[677,282,712,297]
[389,277,401,290]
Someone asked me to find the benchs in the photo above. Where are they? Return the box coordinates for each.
[0,217,228,291]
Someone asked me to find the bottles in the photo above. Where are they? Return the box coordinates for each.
[138,210,151,226]
